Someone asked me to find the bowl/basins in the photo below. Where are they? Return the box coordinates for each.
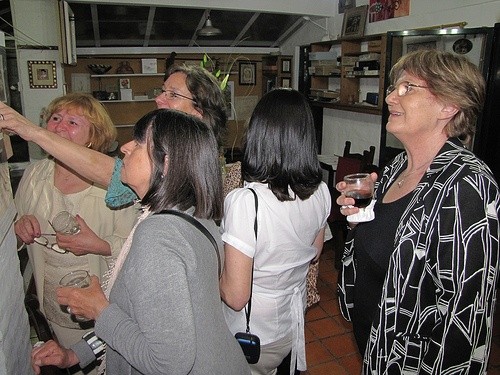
[89,64,112,74]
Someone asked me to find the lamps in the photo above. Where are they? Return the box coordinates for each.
[197,11,221,37]
[302,16,332,41]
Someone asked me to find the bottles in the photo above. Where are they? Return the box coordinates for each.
[116,60,134,74]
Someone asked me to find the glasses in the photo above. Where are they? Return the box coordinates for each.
[386,80,429,96]
[153,87,196,102]
[33,220,67,254]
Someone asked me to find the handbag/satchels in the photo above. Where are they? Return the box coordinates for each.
[235,332,260,364]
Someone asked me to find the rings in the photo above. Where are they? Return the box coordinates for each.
[1,114,4,120]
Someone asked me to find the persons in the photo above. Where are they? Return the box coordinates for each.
[221,88,332,375]
[0,64,253,375]
[335,49,500,375]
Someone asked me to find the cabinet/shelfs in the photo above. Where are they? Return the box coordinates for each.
[307,34,404,116]
[91,72,238,127]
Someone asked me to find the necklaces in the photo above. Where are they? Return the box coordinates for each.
[397,158,433,188]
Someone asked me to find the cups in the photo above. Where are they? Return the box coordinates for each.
[344,173,375,208]
[51,210,81,236]
[58,269,93,322]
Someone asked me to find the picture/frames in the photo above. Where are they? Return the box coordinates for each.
[239,63,257,85]
[341,5,368,38]
[281,59,291,73]
[71,73,91,94]
[27,60,58,89]
[281,77,291,88]
[224,81,235,120]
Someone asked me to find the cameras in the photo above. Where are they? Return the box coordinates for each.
[235,332,261,364]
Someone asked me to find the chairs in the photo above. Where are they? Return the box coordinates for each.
[323,142,375,254]
